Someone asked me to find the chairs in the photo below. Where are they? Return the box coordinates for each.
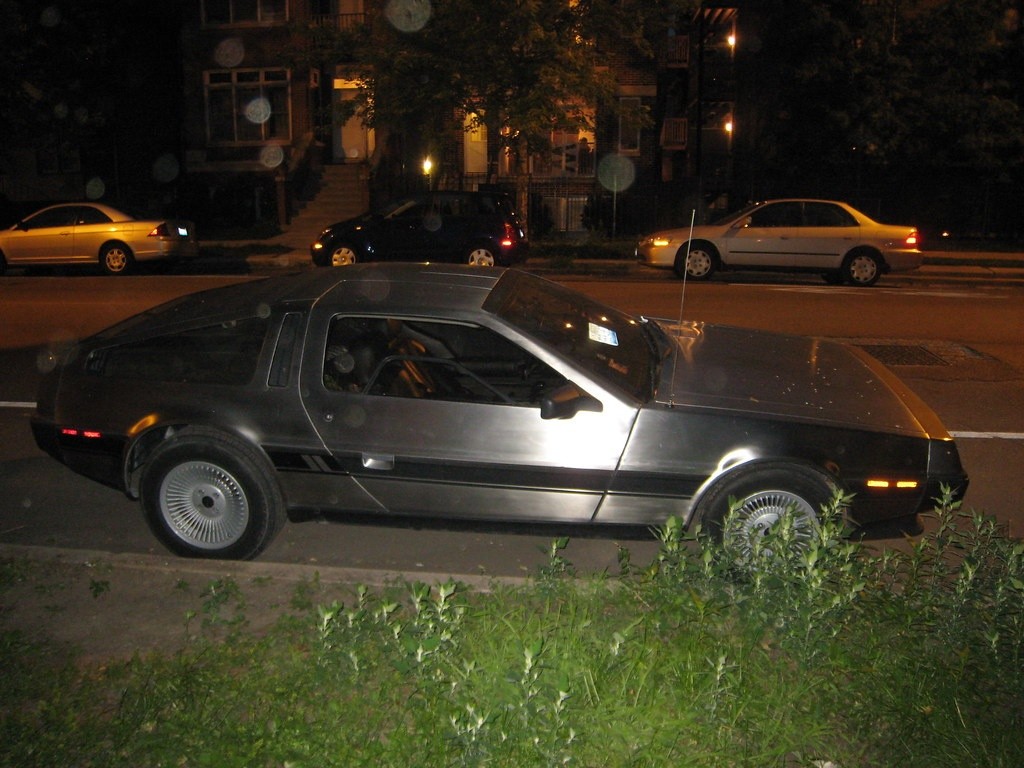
[374,319,469,391]
[348,332,419,399]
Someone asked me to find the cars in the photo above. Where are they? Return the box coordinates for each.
[634,198,924,288]
[309,190,529,269]
[0,200,201,278]
[27,258,973,591]
